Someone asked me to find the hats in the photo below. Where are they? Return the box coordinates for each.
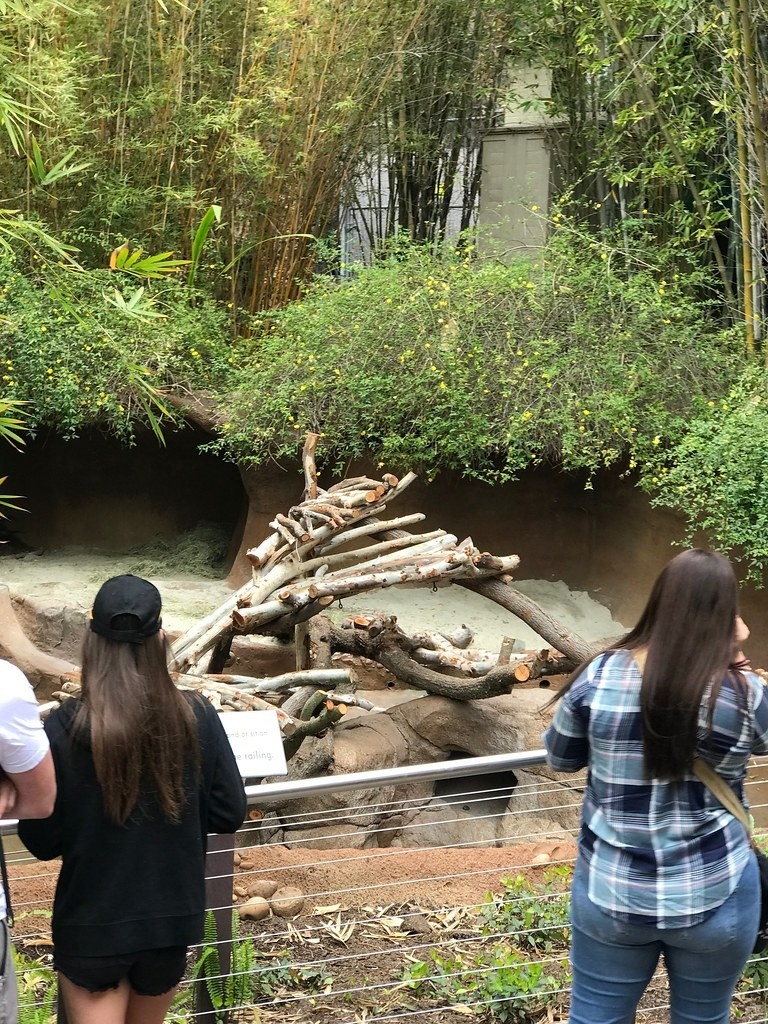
[89,574,163,643]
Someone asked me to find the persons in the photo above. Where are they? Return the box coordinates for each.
[541,546,768,1024]
[16,574,246,1024]
[0,659,57,1024]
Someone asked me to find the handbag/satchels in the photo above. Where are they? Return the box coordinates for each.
[746,834,768,959]
